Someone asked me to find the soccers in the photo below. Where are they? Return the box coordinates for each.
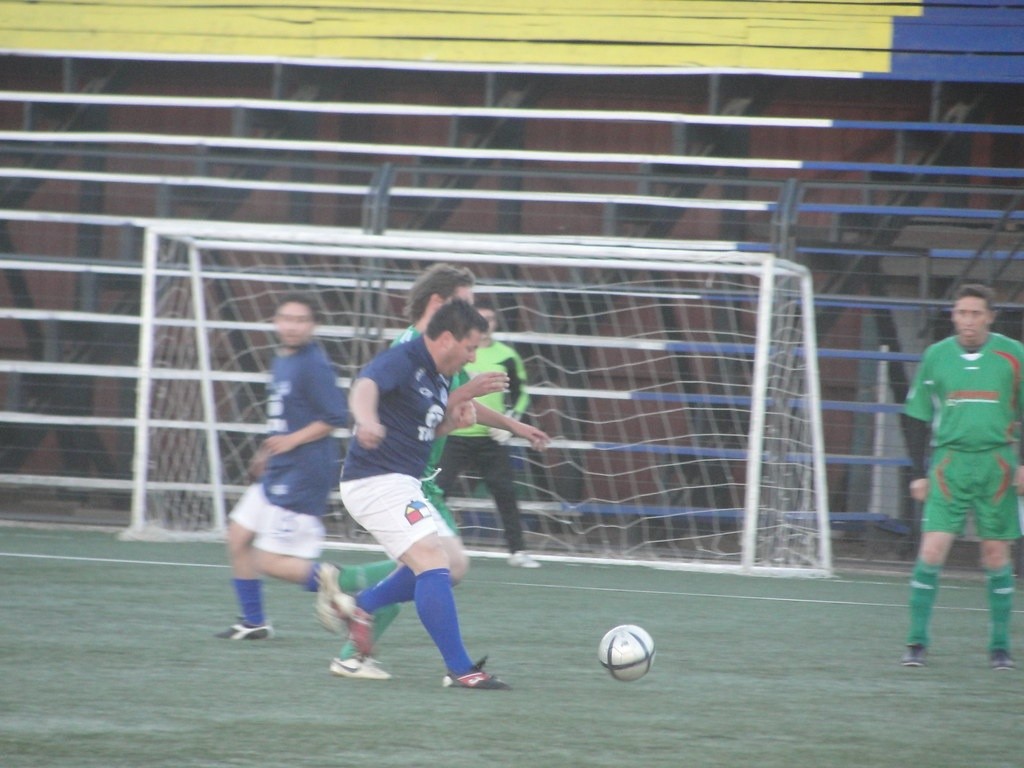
[599,624,655,682]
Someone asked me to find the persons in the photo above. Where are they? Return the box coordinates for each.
[323,261,554,682]
[343,302,515,691]
[895,281,1024,672]
[433,304,549,572]
[211,293,339,645]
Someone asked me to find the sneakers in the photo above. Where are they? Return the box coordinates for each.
[314,562,348,634]
[329,656,392,679]
[331,592,376,658]
[217,619,275,641]
[987,647,1018,670]
[441,669,515,691]
[509,549,542,568]
[899,643,929,667]
